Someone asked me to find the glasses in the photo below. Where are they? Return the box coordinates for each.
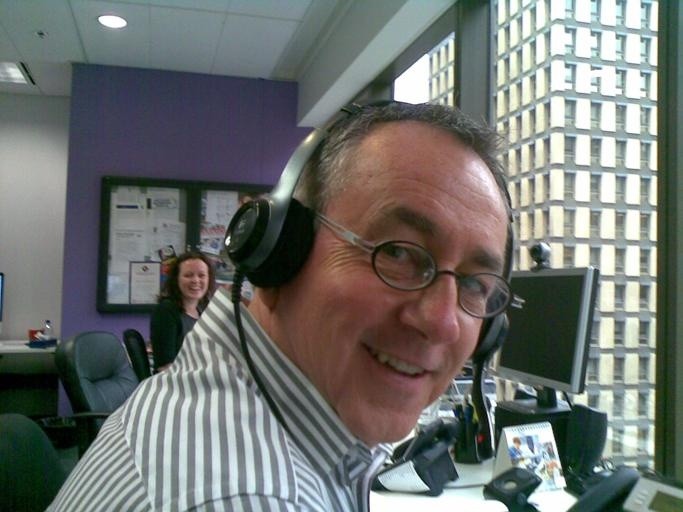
[315,213,528,322]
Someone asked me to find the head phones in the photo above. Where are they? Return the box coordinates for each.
[223,98,515,365]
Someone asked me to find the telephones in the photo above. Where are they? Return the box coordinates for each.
[565,468,683,512]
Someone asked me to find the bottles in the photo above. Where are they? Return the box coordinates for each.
[44,320,54,340]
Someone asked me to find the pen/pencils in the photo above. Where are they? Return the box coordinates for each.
[447,379,475,425]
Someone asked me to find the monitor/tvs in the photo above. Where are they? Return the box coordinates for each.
[487,266,600,418]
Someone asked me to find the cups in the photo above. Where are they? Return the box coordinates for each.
[28,329,43,342]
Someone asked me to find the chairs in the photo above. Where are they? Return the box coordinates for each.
[0,328,154,512]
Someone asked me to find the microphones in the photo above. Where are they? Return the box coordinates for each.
[471,358,496,461]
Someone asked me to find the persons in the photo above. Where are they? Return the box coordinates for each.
[44,98,517,512]
[510,436,536,469]
[150,252,216,375]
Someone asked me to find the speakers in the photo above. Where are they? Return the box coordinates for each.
[514,388,538,403]
[562,402,610,496]
